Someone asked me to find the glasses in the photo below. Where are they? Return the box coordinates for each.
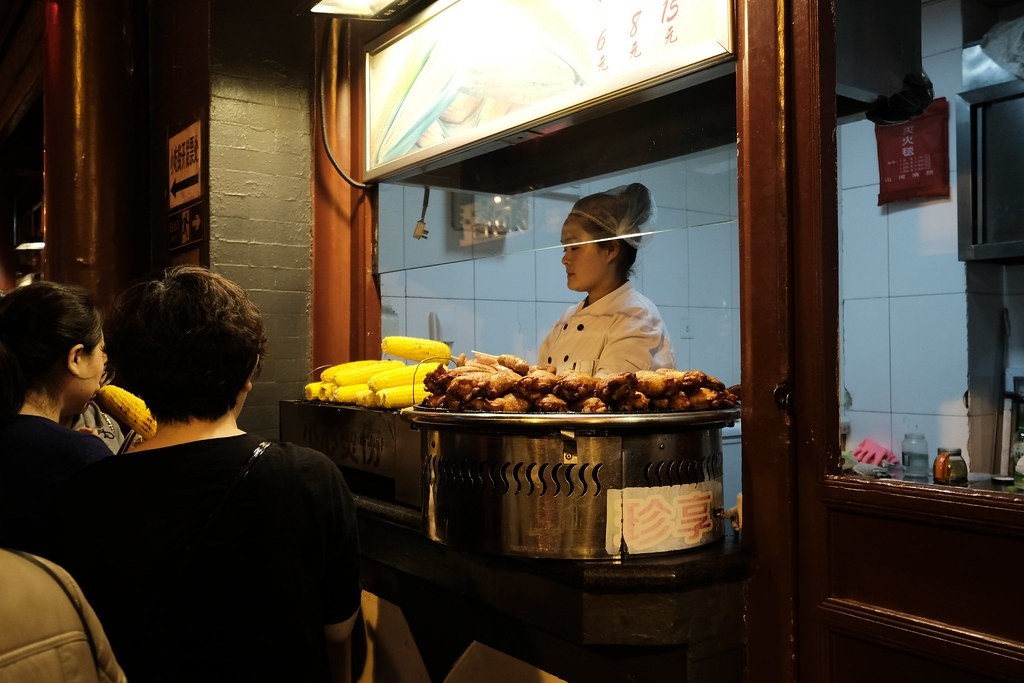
[99,364,117,387]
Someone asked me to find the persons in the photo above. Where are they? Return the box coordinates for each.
[0,547,128,683]
[42,267,362,683]
[536,183,675,377]
[71,363,142,455]
[0,282,115,575]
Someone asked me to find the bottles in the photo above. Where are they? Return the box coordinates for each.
[901,434,930,478]
[1011,427,1024,490]
[932,447,950,484]
[942,448,969,487]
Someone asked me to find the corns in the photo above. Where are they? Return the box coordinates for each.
[97,384,157,440]
[304,336,451,407]
